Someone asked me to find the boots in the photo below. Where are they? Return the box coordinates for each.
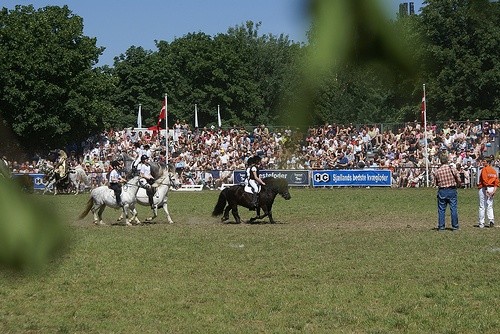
[148,195,155,209]
[132,166,137,176]
[116,193,124,208]
[251,193,260,204]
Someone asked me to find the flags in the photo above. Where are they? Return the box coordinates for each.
[421,97,425,128]
[157,100,166,124]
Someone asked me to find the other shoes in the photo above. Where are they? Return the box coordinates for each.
[490,222,494,228]
[437,227,445,231]
[474,224,482,228]
[452,226,459,230]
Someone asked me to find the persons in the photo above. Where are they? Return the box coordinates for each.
[135,155,158,210]
[108,161,128,207]
[0,116,500,188]
[244,156,266,209]
[478,157,498,229]
[434,153,462,231]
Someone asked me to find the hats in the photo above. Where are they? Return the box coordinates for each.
[131,130,136,133]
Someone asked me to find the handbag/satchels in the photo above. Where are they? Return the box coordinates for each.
[450,166,461,188]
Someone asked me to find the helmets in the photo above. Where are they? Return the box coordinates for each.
[252,155,259,163]
[141,155,149,161]
[143,145,150,149]
[112,161,120,167]
[133,142,140,148]
[0,151,8,158]
[247,157,253,164]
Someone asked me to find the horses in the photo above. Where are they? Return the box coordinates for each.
[212,176,292,224]
[40,165,182,227]
